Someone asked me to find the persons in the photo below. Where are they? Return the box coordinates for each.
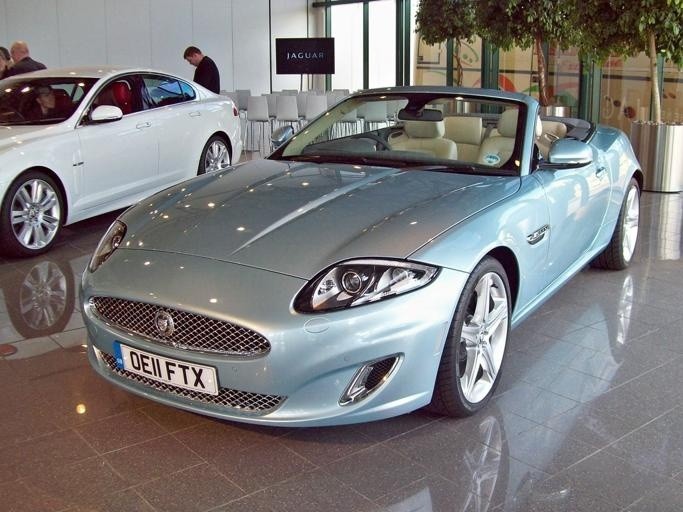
[0,45,11,81]
[181,44,221,96]
[0,39,47,81]
[17,83,68,124]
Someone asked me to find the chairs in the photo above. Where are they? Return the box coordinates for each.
[394,110,567,169]
[98,82,132,113]
[221,90,390,159]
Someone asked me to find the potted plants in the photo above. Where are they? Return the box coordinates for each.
[556,2,683,193]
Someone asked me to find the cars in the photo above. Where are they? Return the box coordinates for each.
[76,87,643,429]
[1,66,242,261]
